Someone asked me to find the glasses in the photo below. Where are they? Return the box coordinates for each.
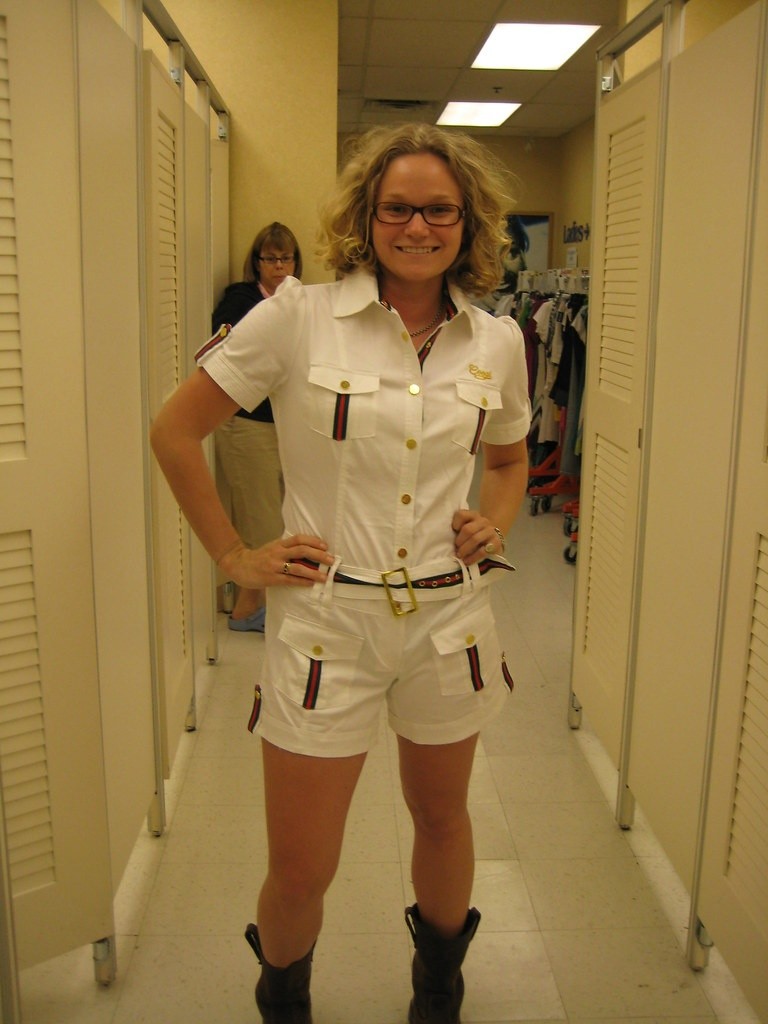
[371,201,466,226]
[256,254,295,264]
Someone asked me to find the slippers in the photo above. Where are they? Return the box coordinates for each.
[229,609,265,632]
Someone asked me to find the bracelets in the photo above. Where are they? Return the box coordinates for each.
[495,528,507,553]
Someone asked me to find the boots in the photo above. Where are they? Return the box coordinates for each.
[405,902,481,1024]
[245,923,317,1024]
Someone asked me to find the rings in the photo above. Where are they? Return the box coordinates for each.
[283,560,292,575]
[484,544,497,553]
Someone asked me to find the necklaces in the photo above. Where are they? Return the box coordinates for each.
[410,306,445,338]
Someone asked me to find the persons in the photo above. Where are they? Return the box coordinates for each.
[213,221,303,633]
[149,120,535,1024]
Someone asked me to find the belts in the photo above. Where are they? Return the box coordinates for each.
[279,530,516,615]
